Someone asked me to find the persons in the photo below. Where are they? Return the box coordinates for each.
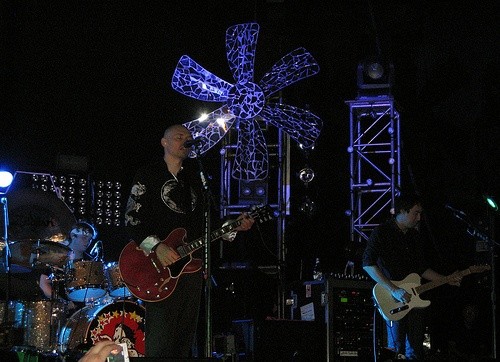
[124,124,255,362]
[361,196,462,362]
[441,301,492,359]
[63,222,95,265]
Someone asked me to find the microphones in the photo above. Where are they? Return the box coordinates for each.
[184,139,202,148]
[444,202,467,216]
[90,241,99,255]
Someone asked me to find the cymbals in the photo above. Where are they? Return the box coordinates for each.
[2,238,73,269]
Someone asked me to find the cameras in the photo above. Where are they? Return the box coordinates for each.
[107,342,130,362]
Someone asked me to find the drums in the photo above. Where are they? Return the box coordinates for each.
[0,300,78,355]
[62,259,107,303]
[103,261,133,297]
[58,294,146,362]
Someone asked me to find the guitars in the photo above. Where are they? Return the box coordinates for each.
[372,263,492,322]
[118,203,274,302]
